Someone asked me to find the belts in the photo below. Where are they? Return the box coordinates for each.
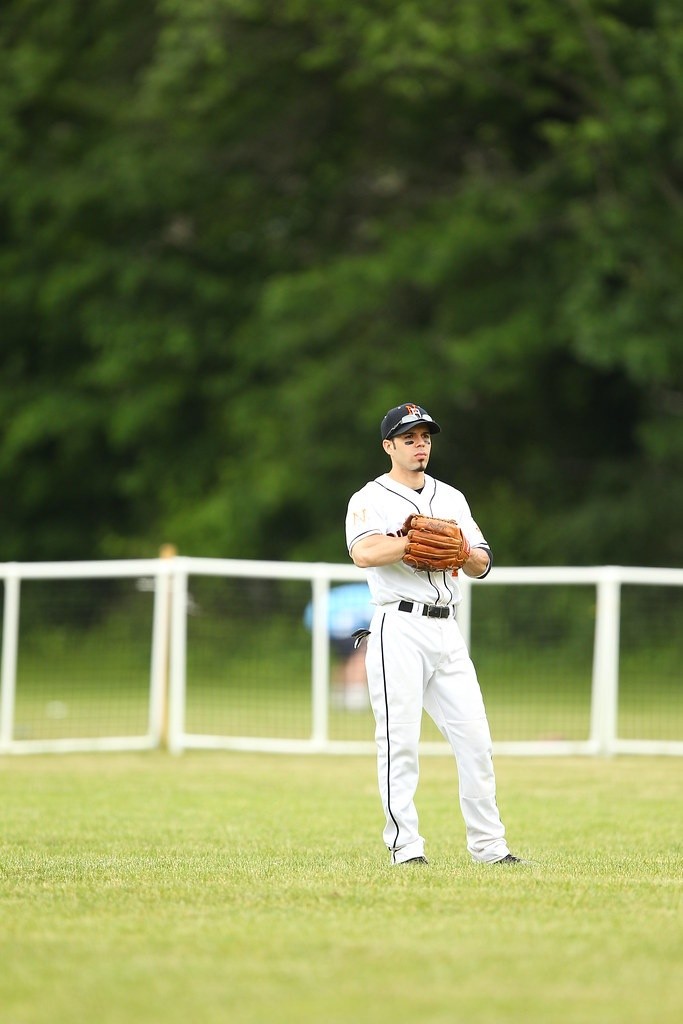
[398,600,454,619]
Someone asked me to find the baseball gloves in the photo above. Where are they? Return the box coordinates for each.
[402,513,470,578]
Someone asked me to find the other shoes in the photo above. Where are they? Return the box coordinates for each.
[494,854,521,865]
[400,856,429,865]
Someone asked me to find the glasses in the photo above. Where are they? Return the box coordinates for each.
[385,414,433,439]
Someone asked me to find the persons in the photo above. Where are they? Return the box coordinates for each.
[346,403,522,866]
[304,584,375,711]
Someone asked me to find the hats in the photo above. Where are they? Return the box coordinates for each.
[381,403,441,441]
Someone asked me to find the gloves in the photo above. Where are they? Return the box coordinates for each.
[351,628,371,650]
